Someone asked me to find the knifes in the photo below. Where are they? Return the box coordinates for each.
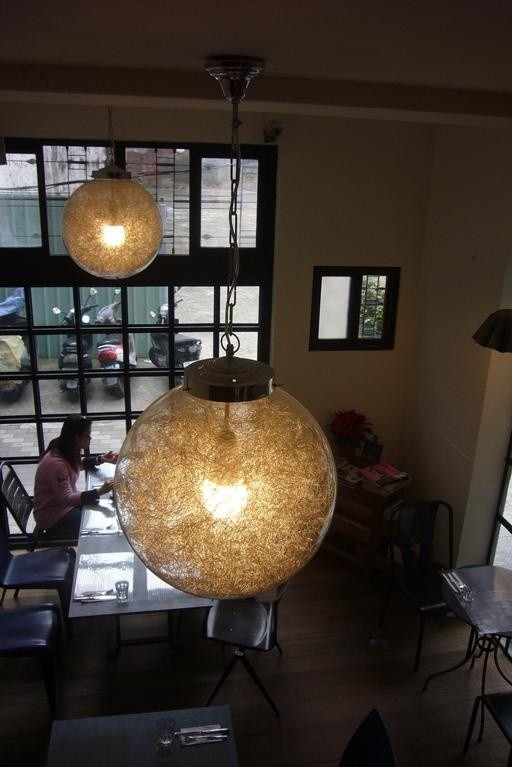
[173,728,229,735]
[73,595,117,600]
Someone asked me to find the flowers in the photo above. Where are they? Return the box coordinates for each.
[331,408,369,446]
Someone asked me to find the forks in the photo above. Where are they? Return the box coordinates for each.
[177,734,229,743]
[73,589,114,596]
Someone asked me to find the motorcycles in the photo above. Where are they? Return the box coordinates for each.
[53,287,98,403]
[81,288,138,400]
[148,297,202,386]
[0,287,39,401]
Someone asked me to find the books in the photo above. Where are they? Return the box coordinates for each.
[334,459,413,500]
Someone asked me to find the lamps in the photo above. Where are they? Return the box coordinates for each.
[111,55,339,601]
[60,106,163,281]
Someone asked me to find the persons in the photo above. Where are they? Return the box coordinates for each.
[32,413,121,536]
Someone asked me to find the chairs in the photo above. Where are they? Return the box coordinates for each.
[463,691,512,767]
[339,708,396,767]
[378,500,454,665]
[199,581,289,718]
[0,602,64,697]
[0,527,76,640]
[0,461,78,552]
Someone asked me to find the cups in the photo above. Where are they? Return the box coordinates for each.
[462,584,476,601]
[115,581,129,603]
[156,716,176,745]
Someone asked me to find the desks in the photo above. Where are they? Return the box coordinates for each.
[67,461,214,619]
[439,564,511,744]
[45,705,239,767]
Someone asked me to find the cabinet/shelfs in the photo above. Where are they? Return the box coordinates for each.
[330,457,413,581]
